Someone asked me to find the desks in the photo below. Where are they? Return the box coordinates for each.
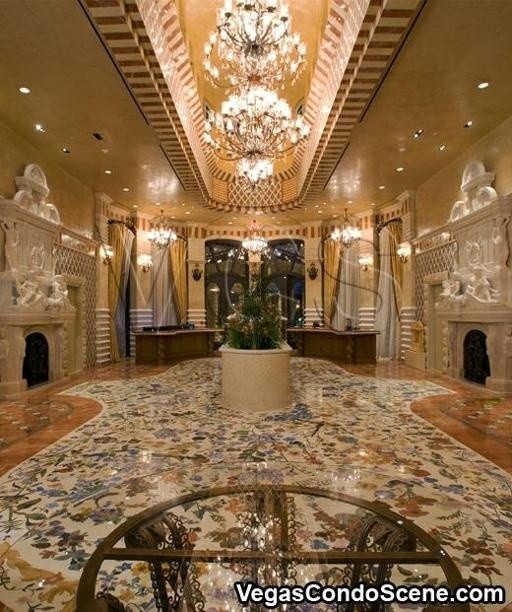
[129,329,225,366]
[285,328,381,364]
[0,461,511,612]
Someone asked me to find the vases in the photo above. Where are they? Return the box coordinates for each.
[217,341,293,412]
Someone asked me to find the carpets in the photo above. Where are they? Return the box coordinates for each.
[1,356,512,612]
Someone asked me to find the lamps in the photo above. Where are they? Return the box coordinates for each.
[201,0,312,193]
[331,207,363,249]
[241,218,269,254]
[146,209,178,250]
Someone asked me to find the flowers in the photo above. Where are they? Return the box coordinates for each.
[222,259,283,350]
